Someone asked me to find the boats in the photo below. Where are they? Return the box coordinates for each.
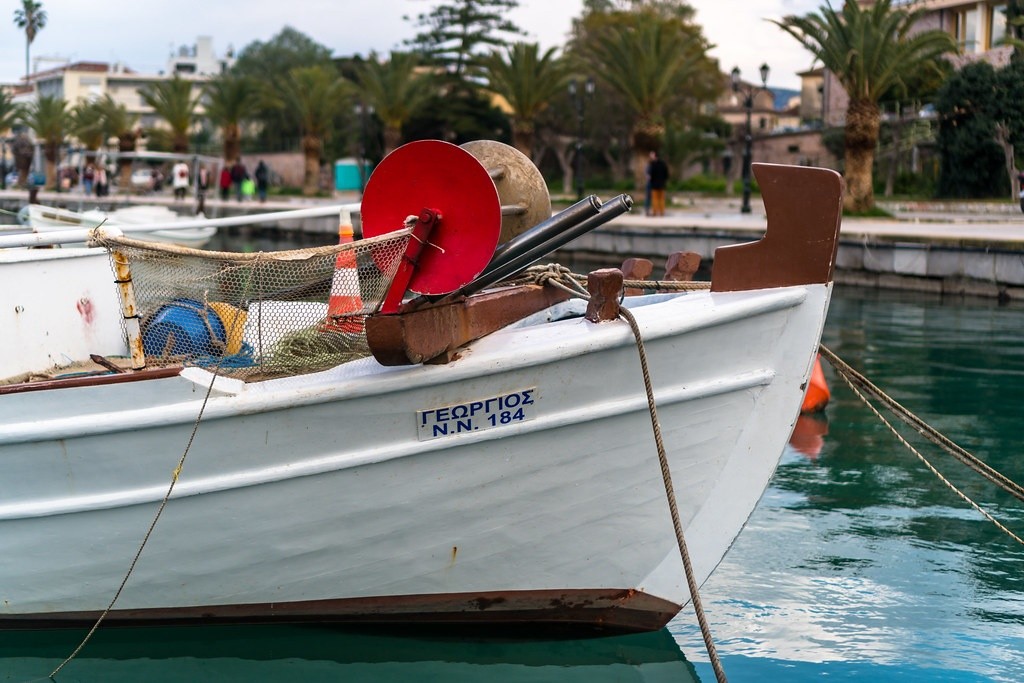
[14,146,226,251]
[0,138,845,630]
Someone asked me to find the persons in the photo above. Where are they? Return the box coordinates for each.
[231,156,249,200]
[170,159,188,199]
[642,150,667,218]
[253,158,270,199]
[219,164,232,199]
[25,169,40,205]
[150,167,163,190]
[79,154,119,196]
[197,162,210,198]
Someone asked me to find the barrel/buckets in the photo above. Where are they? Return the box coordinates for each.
[142,298,227,360]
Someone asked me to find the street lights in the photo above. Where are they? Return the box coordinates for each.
[731,60,772,213]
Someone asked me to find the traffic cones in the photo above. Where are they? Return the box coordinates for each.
[317,201,368,338]
